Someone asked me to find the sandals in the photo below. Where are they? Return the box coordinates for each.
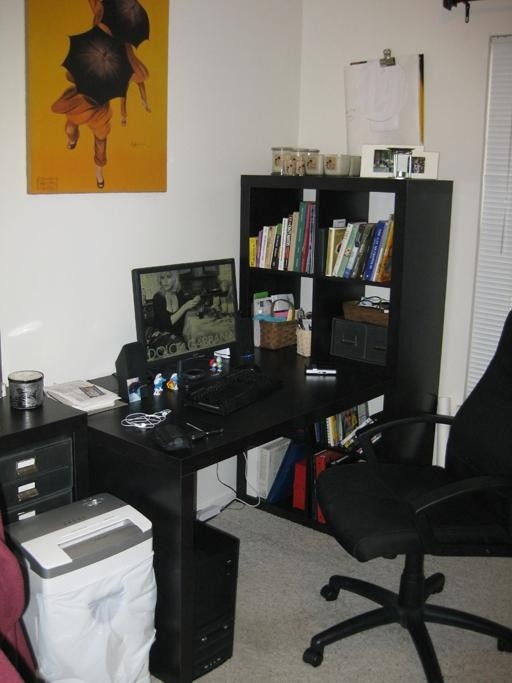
[67,138,77,150]
[97,177,104,188]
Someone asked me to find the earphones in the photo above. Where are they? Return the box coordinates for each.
[161,411,168,417]
[136,423,146,428]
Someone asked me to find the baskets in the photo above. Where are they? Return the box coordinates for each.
[260,299,296,350]
[342,295,390,328]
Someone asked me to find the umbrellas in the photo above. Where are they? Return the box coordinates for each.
[100,0,151,49]
[59,23,136,111]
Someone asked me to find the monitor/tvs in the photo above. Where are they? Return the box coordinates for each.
[132,258,241,388]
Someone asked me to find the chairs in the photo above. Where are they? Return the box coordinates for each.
[302,308,512,683]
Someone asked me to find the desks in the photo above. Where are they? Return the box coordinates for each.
[87,344,385,683]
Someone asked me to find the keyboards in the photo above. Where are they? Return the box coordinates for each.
[183,369,279,416]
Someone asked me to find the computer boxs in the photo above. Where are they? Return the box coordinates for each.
[192,517,240,678]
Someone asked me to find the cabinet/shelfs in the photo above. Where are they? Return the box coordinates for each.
[236,174,454,559]
[0,395,88,525]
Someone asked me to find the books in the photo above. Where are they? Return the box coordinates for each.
[248,198,317,275]
[317,402,383,453]
[325,215,394,284]
[44,382,128,416]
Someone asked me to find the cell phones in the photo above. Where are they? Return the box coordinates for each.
[305,361,338,376]
[186,419,224,435]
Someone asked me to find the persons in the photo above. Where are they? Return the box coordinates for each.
[153,270,201,333]
[88,0,154,128]
[50,66,114,190]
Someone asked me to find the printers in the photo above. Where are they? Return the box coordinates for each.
[5,493,154,594]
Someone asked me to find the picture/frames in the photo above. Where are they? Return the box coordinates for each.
[131,257,241,369]
